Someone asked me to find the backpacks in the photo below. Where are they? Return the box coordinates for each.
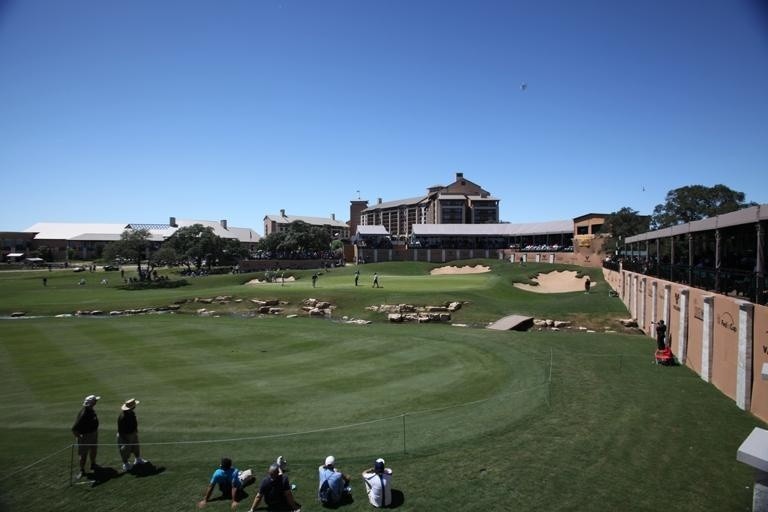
[319,473,332,505]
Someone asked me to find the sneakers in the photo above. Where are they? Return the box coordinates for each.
[134,457,148,465]
[122,462,133,470]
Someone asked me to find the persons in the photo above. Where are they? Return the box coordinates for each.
[71,394,103,479]
[361,457,393,508]
[372,272,381,289]
[651,319,667,351]
[40,275,49,288]
[318,456,353,509]
[420,238,708,287]
[120,247,371,290]
[248,464,302,511]
[115,398,150,471]
[196,458,254,508]
[583,278,594,295]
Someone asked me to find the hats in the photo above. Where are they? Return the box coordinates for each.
[325,455,335,466]
[375,458,386,473]
[121,398,141,411]
[81,395,101,406]
